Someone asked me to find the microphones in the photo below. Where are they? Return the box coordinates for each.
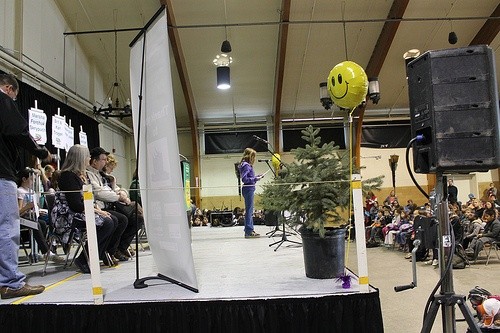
[253,135,268,144]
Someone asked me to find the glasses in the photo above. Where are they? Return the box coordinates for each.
[97,158,107,161]
[6,86,17,101]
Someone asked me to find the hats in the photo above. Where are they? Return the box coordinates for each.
[90,147,110,156]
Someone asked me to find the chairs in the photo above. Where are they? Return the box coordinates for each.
[18,170,148,277]
[455,225,500,266]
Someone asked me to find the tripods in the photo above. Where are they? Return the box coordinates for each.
[258,141,302,251]
[421,174,483,333]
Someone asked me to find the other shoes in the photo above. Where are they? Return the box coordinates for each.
[404,253,412,258]
[245,232,260,238]
[103,255,118,265]
[75,258,90,273]
[115,250,128,261]
[49,254,64,262]
[464,247,474,253]
[424,259,432,265]
[124,250,135,257]
[468,255,475,260]
[253,231,259,235]
[432,259,439,265]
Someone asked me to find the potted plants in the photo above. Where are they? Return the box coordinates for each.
[256,125,385,279]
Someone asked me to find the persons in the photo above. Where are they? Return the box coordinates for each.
[16,144,144,273]
[345,178,500,326]
[237,148,264,239]
[0,73,52,299]
[192,207,264,227]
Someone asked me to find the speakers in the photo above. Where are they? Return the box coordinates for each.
[405,46,499,174]
[211,213,234,227]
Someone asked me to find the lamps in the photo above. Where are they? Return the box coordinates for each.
[319,82,334,110]
[221,26,232,52]
[449,20,457,44]
[216,65,231,90]
[369,77,380,105]
[235,162,242,201]
[390,153,399,188]
[93,32,133,120]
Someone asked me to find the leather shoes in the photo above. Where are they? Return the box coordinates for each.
[0,284,45,298]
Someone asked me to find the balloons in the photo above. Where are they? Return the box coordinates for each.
[327,61,369,112]
[272,153,280,168]
